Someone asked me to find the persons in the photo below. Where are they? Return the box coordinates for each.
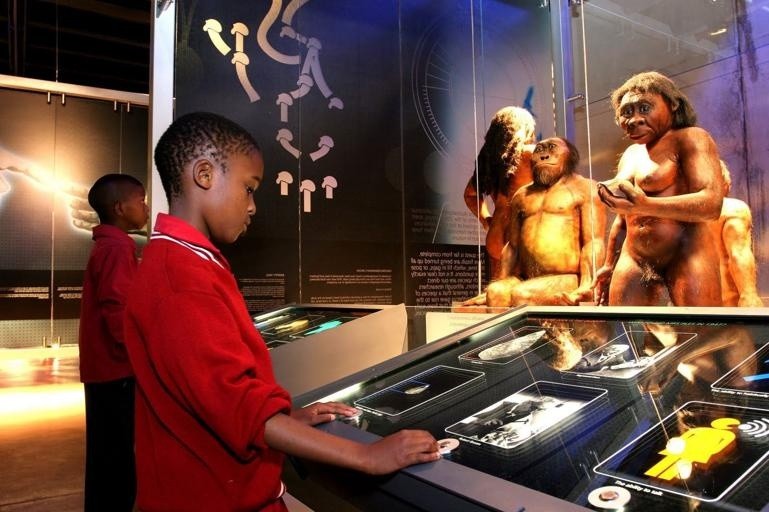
[124,111,441,512]
[79,173,150,512]
[512,321,758,438]
